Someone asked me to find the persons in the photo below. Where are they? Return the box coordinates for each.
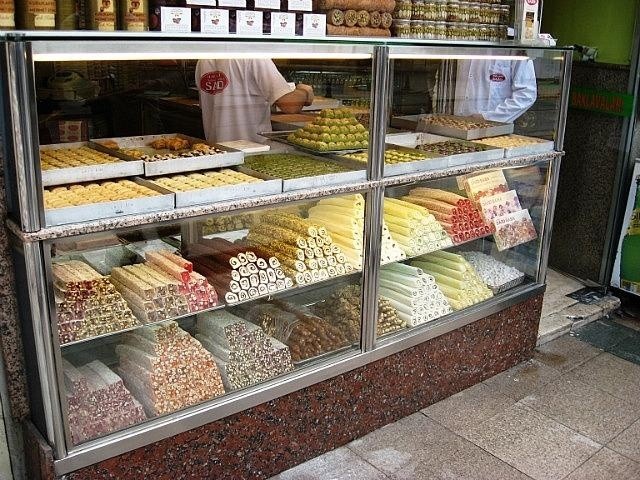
[194,56,314,145]
[431,58,537,124]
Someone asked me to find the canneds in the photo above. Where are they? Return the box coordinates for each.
[396,0,510,42]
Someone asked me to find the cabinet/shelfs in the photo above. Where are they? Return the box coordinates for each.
[6,38,574,479]
